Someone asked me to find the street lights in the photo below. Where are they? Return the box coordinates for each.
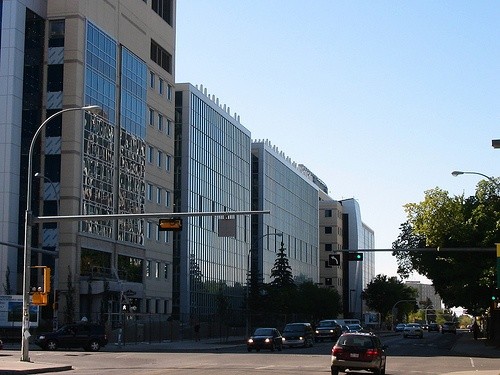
[350,289,356,319]
[35,173,60,332]
[244,232,283,337]
[20,104,102,361]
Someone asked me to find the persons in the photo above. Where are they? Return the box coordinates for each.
[472,322,480,340]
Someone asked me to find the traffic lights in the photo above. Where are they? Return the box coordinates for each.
[44,267,55,294]
[347,253,363,261]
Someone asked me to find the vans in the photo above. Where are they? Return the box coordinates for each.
[281,323,315,348]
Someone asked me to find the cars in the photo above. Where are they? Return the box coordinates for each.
[441,322,456,335]
[428,324,440,332]
[348,324,364,333]
[342,326,350,334]
[330,332,389,375]
[403,323,424,338]
[247,328,283,352]
[422,324,428,330]
[396,323,406,332]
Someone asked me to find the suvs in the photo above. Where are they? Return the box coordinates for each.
[35,323,108,352]
[315,320,341,343]
